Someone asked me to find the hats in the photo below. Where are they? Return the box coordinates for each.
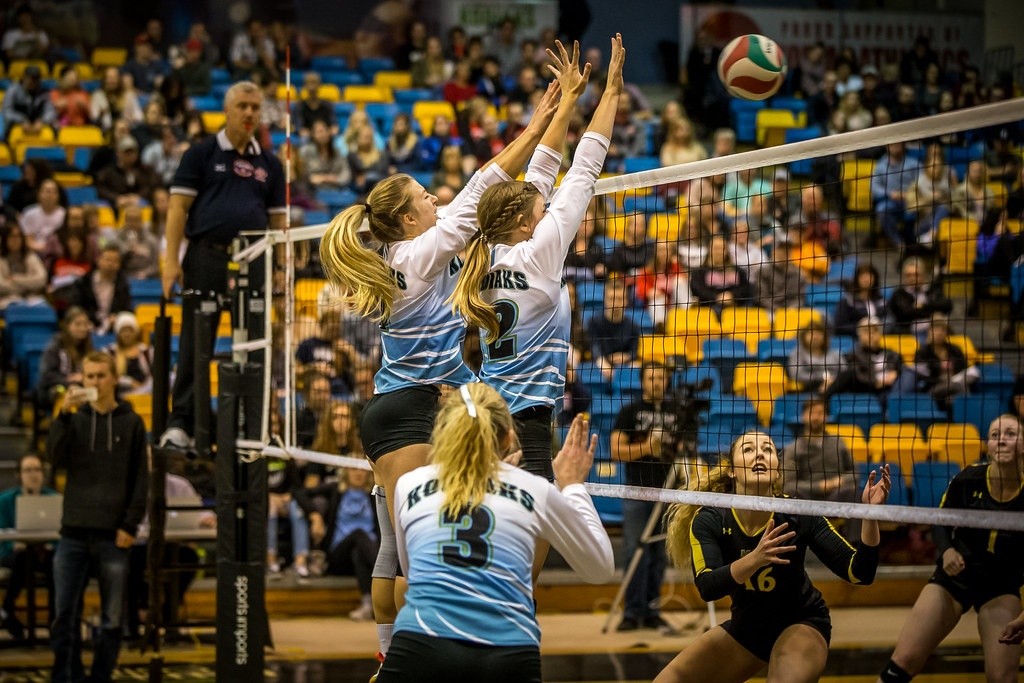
[117,135,138,152]
[113,310,139,336]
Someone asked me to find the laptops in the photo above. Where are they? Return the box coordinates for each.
[165,497,203,531]
[16,495,64,532]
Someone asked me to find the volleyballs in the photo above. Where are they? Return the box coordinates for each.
[716,33,790,102]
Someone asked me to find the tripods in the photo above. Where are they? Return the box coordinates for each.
[602,432,721,637]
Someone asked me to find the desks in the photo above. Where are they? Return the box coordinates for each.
[0,527,218,650]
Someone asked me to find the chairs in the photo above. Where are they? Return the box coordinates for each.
[0,48,1024,549]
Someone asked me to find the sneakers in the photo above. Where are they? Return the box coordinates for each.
[159,426,196,452]
[369,651,386,682]
[614,604,670,637]
[347,593,374,623]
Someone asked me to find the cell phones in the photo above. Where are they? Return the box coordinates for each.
[72,386,98,401]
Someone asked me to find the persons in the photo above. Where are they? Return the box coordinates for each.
[607,361,694,634]
[43,353,150,683]
[445,30,626,613]
[0,209,384,621]
[159,81,291,449]
[319,79,562,683]
[124,475,206,648]
[0,8,564,223]
[565,28,1024,420]
[647,431,892,683]
[877,414,1024,683]
[0,453,66,643]
[778,395,860,537]
[374,383,615,683]
